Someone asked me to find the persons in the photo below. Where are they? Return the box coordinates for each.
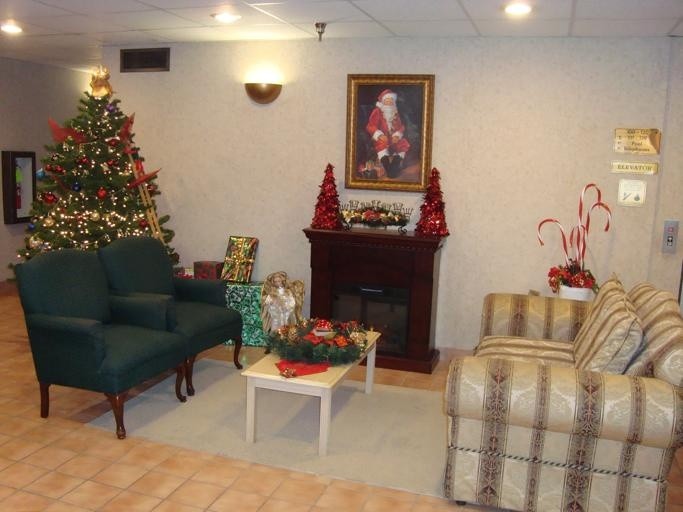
[261,270,297,334]
[365,89,411,178]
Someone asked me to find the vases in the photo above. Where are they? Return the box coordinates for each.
[558,285,591,301]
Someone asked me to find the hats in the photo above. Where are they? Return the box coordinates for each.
[377,89,398,102]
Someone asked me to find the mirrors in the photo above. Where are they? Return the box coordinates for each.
[1,150,36,224]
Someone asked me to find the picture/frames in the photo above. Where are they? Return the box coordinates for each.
[344,73,435,193]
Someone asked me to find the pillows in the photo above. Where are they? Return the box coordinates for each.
[570,271,644,378]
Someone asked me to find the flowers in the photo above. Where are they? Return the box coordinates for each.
[339,205,408,227]
[547,258,599,295]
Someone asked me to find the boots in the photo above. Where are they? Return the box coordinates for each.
[380,155,401,178]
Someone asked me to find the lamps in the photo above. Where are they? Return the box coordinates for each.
[241,70,282,104]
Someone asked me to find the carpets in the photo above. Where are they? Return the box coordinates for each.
[81,356,448,502]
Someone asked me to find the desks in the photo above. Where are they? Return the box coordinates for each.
[239,330,381,457]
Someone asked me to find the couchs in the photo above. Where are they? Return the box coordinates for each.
[441,280,682,512]
